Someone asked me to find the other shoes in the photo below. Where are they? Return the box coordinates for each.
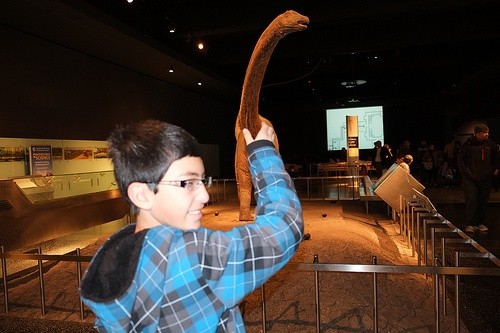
[478,224,488,231]
[465,225,479,233]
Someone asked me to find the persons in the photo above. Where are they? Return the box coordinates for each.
[288,137,471,189]
[459,124,499,234]
[79,114,303,333]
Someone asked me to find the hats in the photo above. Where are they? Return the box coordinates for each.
[474,124,489,132]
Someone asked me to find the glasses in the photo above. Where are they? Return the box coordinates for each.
[146,177,212,191]
